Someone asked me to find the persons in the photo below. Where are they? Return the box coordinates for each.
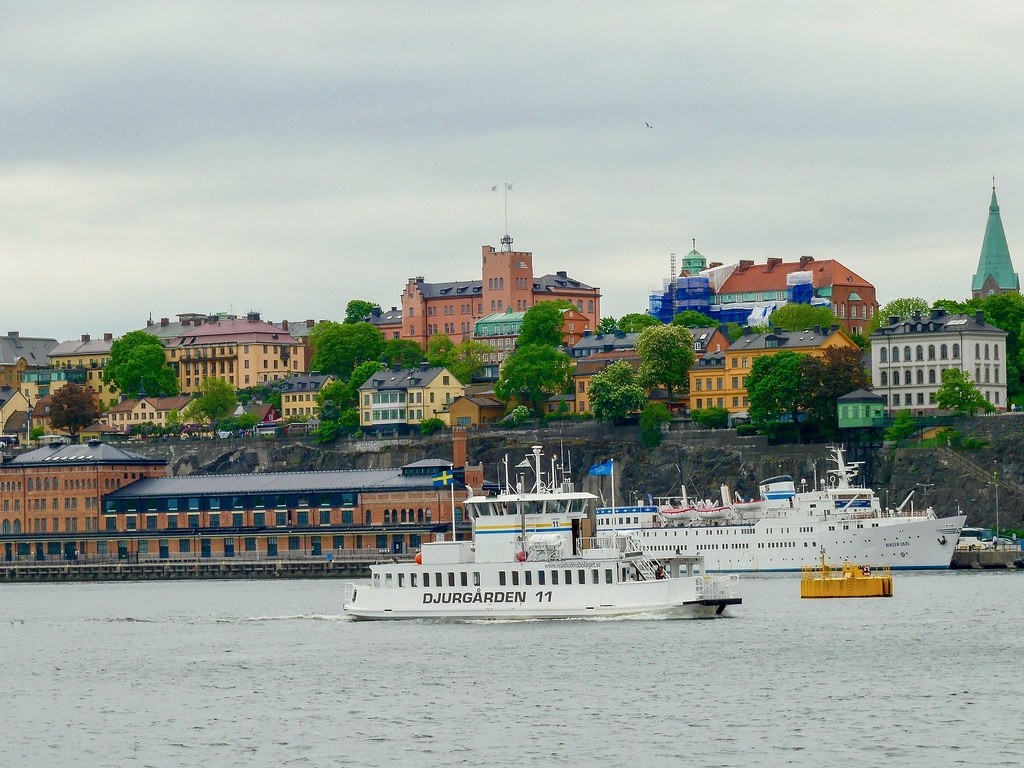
[993,534,998,549]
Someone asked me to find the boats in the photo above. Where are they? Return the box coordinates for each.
[594,448,970,572]
[342,443,743,622]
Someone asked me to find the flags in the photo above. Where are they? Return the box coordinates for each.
[515,458,530,467]
[432,470,453,486]
[590,461,612,475]
